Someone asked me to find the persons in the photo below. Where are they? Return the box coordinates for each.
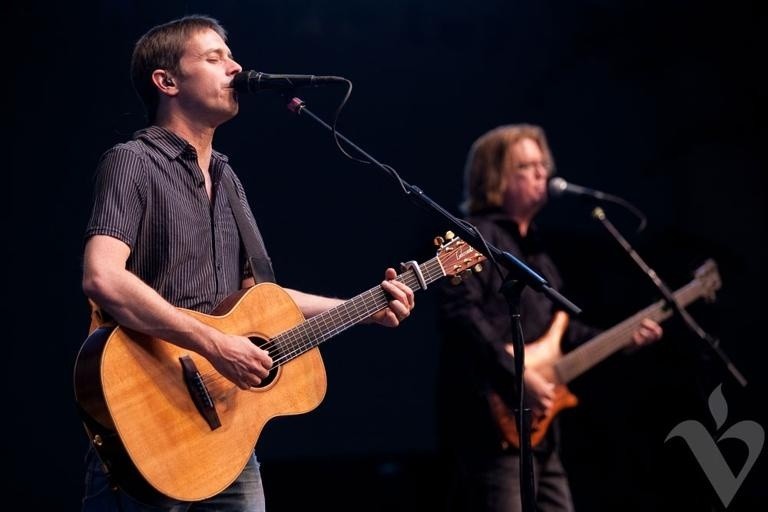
[437,123,663,512]
[81,13,414,512]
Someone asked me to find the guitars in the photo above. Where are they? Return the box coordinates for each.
[482,259,723,454]
[74,228,487,508]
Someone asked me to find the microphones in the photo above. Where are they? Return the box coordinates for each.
[232,69,347,97]
[547,176,645,221]
